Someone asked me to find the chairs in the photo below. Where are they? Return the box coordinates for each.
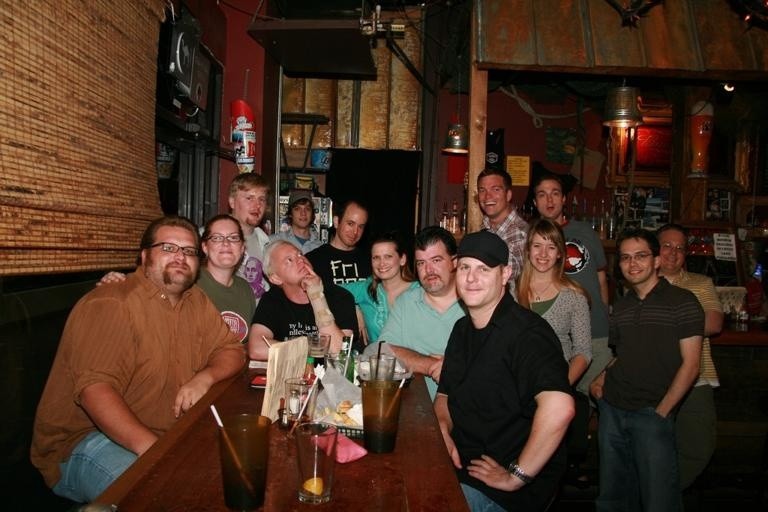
[512,391,590,512]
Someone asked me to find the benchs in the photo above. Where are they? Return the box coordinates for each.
[0,268,140,512]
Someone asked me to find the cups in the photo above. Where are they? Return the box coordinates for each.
[293,420,339,506]
[361,379,405,456]
[448,124,467,148]
[216,413,272,512]
[276,330,351,429]
[366,352,398,380]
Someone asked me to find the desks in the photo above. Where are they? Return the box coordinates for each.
[87,353,470,512]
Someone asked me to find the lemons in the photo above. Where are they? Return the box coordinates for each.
[303,478,323,496]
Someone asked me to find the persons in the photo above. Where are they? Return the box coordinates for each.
[30,166,726,511]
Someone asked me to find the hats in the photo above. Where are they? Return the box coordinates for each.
[450,230,510,268]
[288,190,315,201]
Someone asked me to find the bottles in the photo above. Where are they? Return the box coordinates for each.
[339,336,355,385]
[724,303,751,325]
[438,197,468,234]
[512,194,617,237]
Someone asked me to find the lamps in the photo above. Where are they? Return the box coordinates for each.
[439,43,470,155]
[602,75,644,130]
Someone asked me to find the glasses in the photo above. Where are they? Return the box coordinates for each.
[201,233,244,244]
[145,242,201,258]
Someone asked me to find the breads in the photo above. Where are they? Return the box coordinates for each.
[336,400,351,413]
[343,419,358,426]
[332,413,349,423]
